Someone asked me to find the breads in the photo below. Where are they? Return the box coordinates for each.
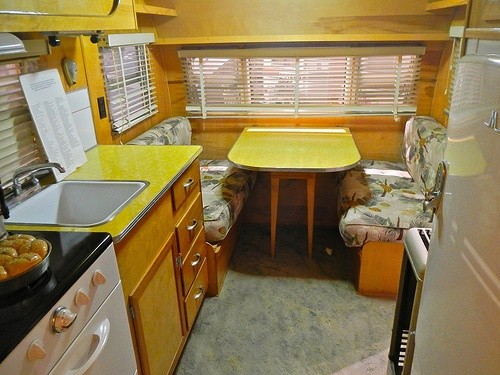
[0,233,49,281]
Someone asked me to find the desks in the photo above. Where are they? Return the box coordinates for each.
[225,125,362,257]
[0,143,210,375]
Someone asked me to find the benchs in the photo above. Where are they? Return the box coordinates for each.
[123,115,253,298]
[338,114,450,295]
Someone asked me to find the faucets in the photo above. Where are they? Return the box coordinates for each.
[12,161,66,197]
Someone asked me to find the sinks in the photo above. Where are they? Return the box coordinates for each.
[0,178,151,228]
